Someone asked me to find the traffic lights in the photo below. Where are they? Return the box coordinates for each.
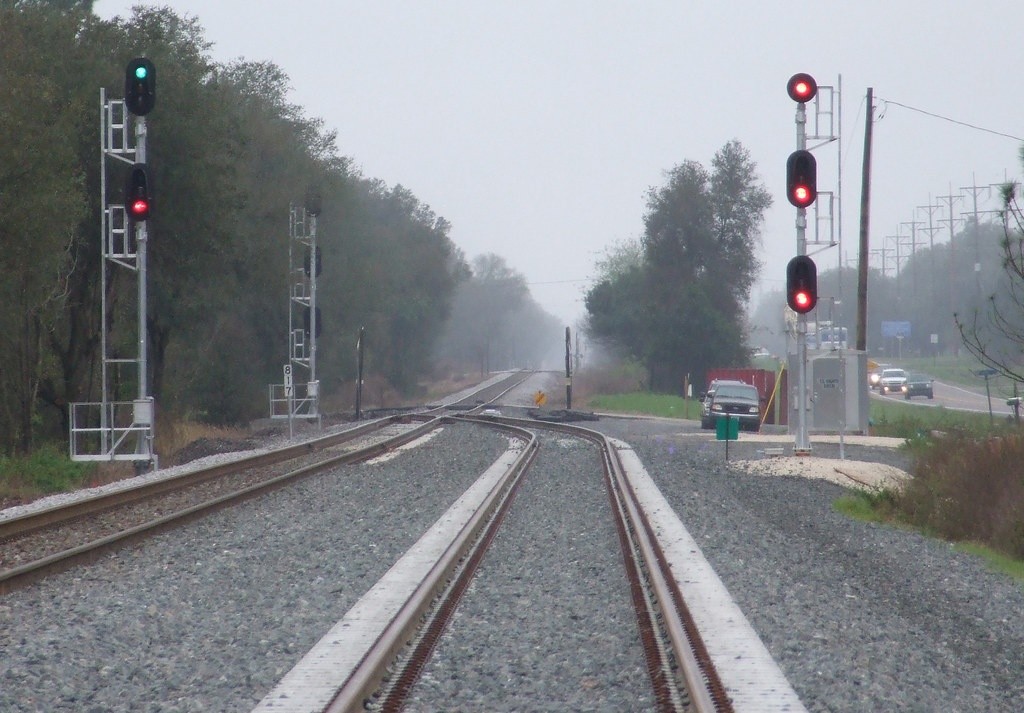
[123,58,159,117]
[784,255,818,313]
[781,67,817,105]
[124,167,149,221]
[784,149,818,208]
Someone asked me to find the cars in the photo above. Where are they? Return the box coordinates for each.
[900,373,935,401]
[879,369,904,394]
[705,382,762,434]
[700,376,745,431]
[749,344,773,359]
[868,360,881,385]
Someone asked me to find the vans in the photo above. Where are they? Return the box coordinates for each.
[818,324,851,351]
